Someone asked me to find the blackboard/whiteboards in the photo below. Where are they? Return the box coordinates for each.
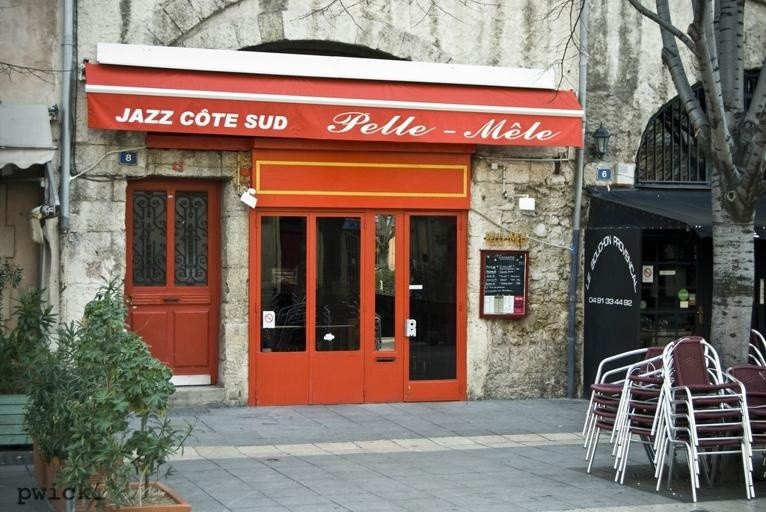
[480,250,529,318]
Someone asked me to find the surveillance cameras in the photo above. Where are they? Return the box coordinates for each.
[241,194,258,209]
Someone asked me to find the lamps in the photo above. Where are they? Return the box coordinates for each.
[592,121,611,161]
[235,153,258,209]
[502,164,536,211]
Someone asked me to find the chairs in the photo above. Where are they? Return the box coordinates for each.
[581,337,765,505]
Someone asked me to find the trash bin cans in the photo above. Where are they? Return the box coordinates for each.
[641,311,693,358]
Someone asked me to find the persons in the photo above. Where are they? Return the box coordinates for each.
[271,278,293,313]
